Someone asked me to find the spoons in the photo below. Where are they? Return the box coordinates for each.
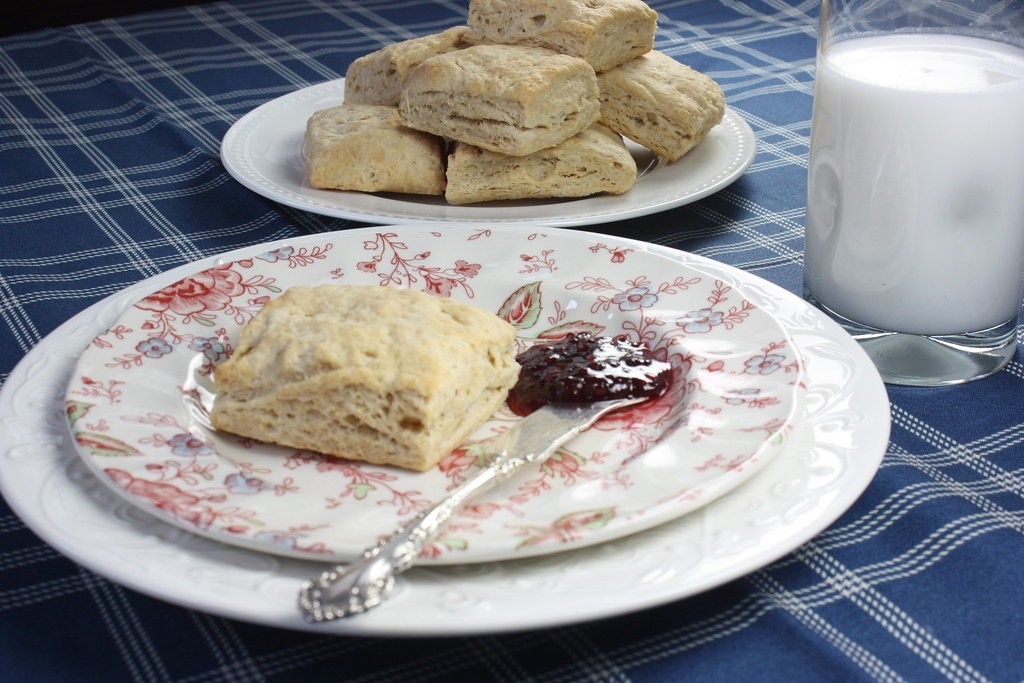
[295,376,670,624]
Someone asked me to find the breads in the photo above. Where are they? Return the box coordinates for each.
[305,0,726,205]
[208,283,523,472]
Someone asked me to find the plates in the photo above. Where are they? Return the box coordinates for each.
[0,228,891,638]
[219,77,757,227]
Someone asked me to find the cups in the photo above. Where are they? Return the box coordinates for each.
[803,0,1024,386]
